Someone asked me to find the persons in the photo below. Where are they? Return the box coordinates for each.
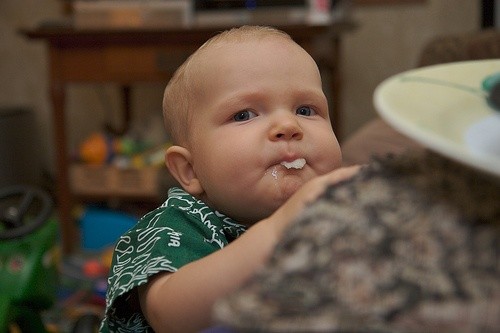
[98,25,366,332]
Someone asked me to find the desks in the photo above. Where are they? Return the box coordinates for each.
[15,17,364,269]
